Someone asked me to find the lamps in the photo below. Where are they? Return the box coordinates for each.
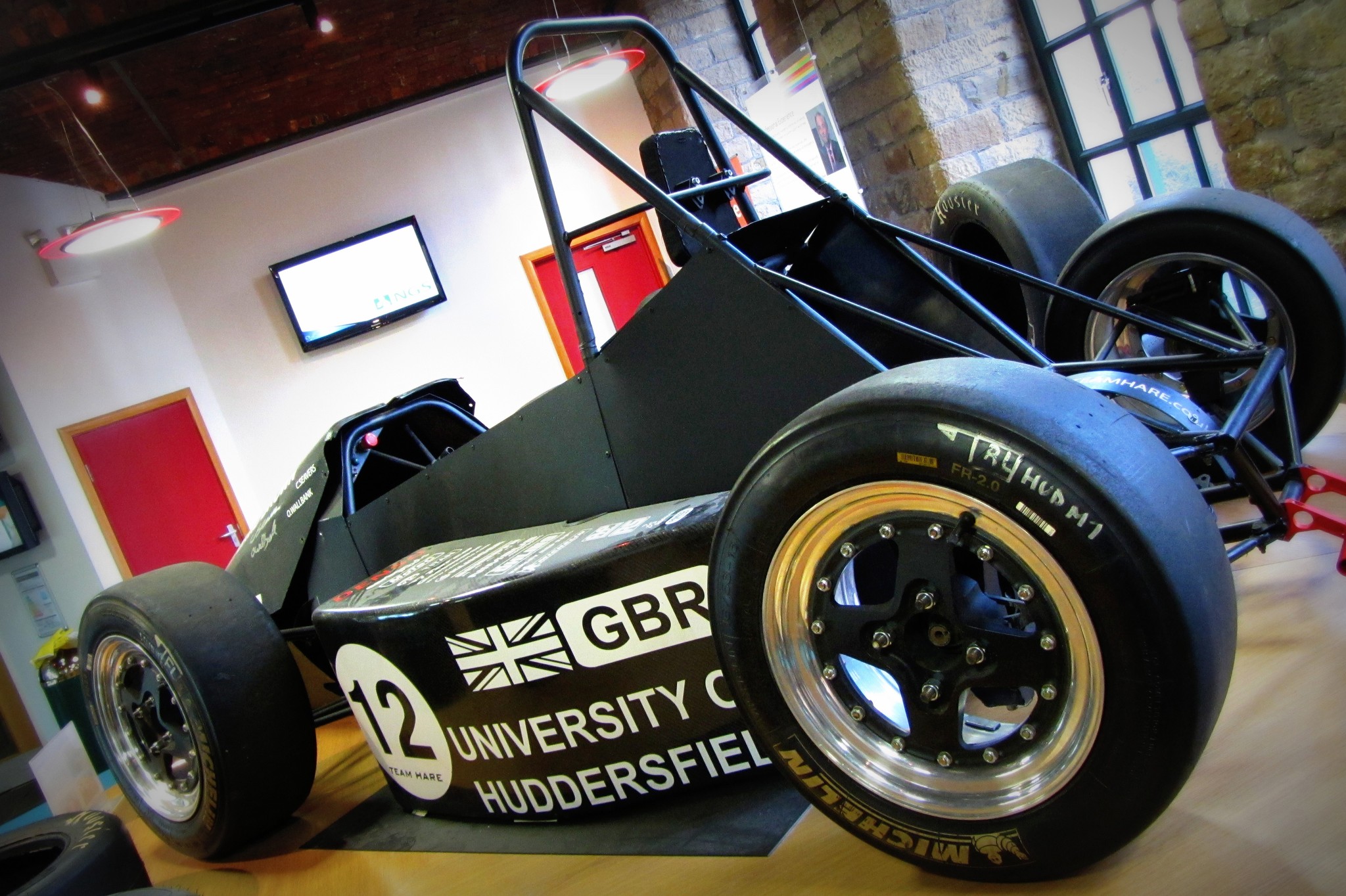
[30,83,181,260]
[532,0,644,101]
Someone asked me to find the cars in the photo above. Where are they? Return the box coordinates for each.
[26,14,1346,886]
